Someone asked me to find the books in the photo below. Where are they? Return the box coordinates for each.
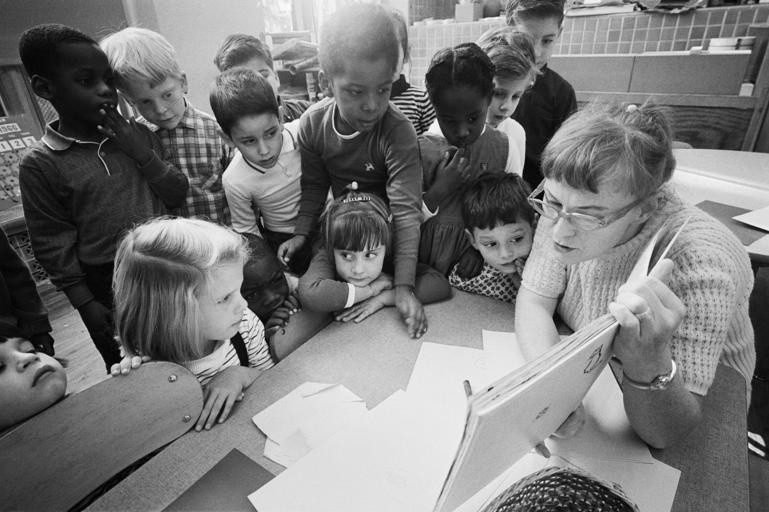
[432,214,691,512]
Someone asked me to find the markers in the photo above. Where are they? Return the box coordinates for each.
[463,380,473,397]
[536,442,550,458]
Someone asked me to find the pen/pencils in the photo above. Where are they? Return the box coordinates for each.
[445,135,467,165]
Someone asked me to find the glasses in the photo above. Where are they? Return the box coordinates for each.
[526,168,663,230]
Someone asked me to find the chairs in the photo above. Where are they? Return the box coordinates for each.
[269,305,333,366]
[2,361,203,512]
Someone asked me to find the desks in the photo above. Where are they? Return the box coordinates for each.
[661,149,768,276]
[79,269,756,511]
